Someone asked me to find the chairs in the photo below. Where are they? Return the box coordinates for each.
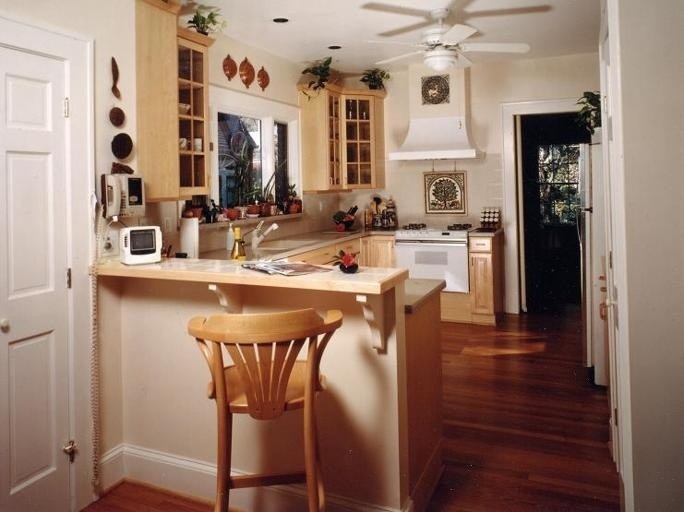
[186,307,344,511]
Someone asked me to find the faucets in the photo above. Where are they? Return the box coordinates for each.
[249,220,278,261]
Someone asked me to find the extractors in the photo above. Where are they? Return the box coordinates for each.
[388,51,485,161]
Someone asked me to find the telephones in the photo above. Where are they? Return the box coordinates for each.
[101,173,147,221]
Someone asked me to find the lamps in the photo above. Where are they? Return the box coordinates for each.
[422,45,457,72]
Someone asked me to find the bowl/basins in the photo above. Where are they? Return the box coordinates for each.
[178,102,191,114]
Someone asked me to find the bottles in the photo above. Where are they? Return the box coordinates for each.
[231,226,247,262]
[372,208,397,229]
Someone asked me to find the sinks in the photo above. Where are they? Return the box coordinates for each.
[260,239,331,248]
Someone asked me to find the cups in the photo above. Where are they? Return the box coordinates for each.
[270,205,277,216]
[194,138,202,152]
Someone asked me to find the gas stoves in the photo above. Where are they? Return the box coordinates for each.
[395,224,479,242]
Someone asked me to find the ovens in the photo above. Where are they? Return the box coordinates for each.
[393,242,470,295]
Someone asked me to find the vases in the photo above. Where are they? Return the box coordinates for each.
[340,264,358,274]
[187,207,204,220]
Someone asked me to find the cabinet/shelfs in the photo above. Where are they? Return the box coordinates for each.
[468,230,504,327]
[273,235,395,270]
[296,82,384,195]
[136,0,216,203]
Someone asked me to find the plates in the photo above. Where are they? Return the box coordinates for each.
[245,213,260,218]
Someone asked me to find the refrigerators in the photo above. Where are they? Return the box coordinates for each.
[575,142,610,386]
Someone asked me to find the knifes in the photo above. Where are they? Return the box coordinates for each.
[347,205,358,216]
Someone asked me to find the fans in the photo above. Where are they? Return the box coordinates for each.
[366,7,531,67]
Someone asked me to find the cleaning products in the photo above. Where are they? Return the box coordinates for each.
[226,223,234,251]
[230,228,246,260]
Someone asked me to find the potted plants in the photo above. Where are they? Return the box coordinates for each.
[285,184,299,213]
[573,90,601,144]
[359,68,389,89]
[301,56,332,101]
[205,199,218,223]
[230,141,277,216]
[186,7,223,36]
